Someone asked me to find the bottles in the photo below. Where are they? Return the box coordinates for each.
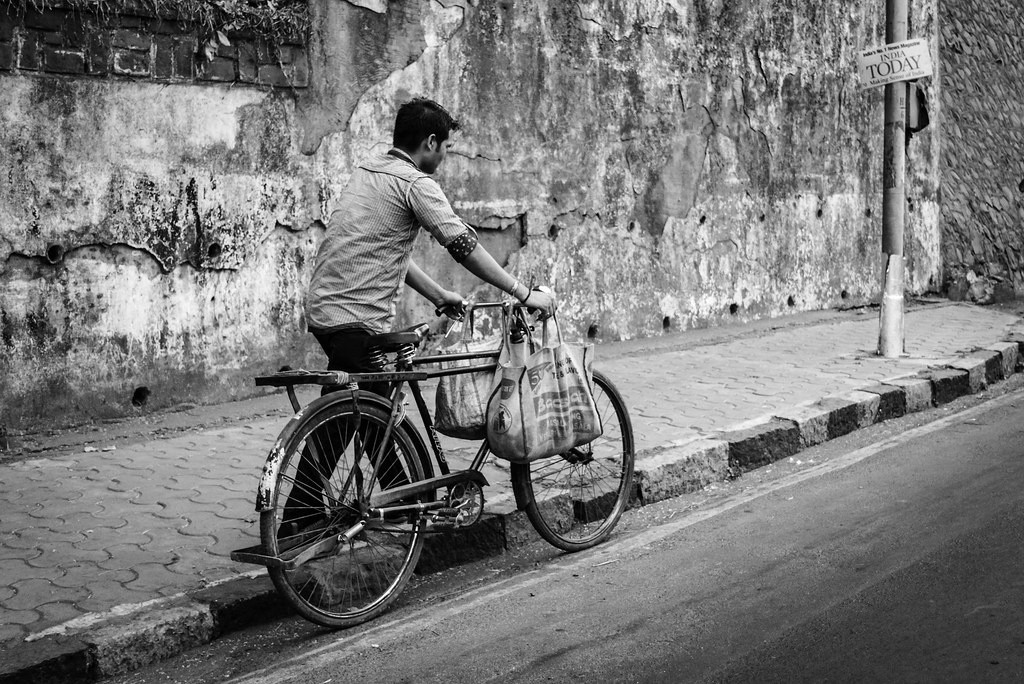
[510,324,529,364]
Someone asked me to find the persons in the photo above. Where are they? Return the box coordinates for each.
[275,97,560,542]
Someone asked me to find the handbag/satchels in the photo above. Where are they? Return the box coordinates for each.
[434,301,504,440]
[485,300,603,465]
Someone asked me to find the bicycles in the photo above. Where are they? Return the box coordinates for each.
[228,287,636,630]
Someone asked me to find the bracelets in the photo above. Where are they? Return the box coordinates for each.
[520,289,531,303]
[509,280,519,295]
